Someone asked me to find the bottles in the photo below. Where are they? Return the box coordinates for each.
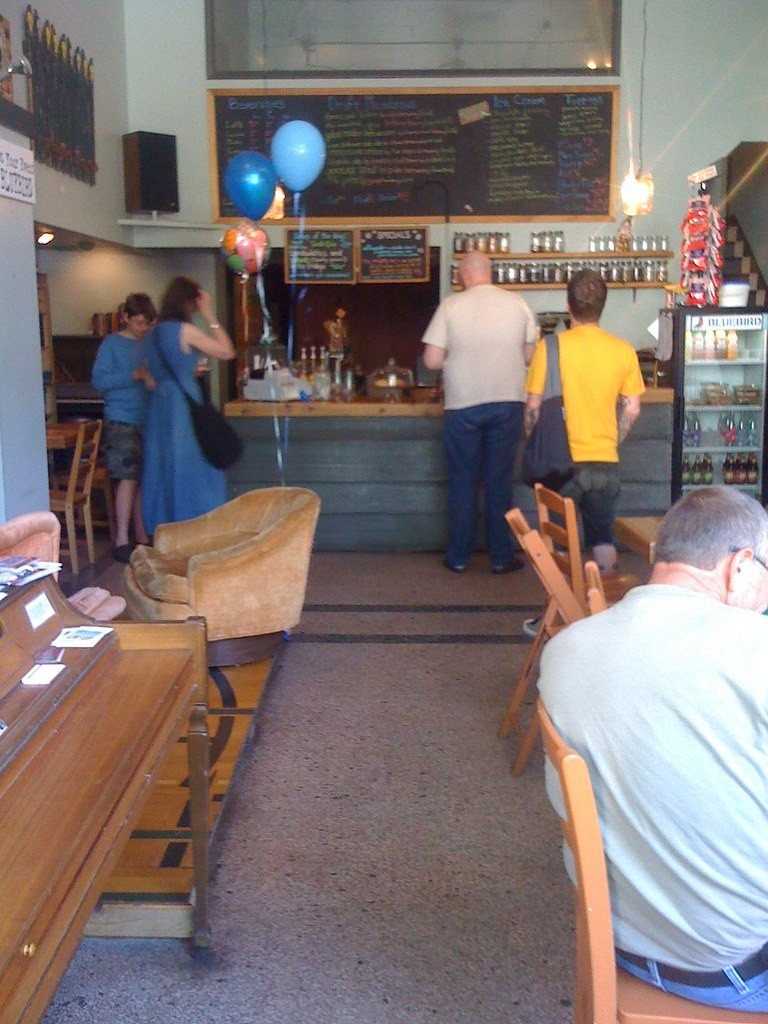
[682,452,759,486]
[249,346,365,399]
[450,229,669,282]
[681,411,760,448]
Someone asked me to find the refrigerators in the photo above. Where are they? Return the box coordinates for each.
[674,305,768,510]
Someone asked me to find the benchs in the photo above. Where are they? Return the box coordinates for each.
[52,454,119,544]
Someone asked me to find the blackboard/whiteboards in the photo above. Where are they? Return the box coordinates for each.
[283,226,357,284]
[357,225,429,282]
[206,85,621,223]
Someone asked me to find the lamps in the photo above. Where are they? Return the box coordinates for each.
[621,0,655,216]
[260,0,286,221]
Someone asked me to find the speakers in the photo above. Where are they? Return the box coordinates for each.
[122,131,179,217]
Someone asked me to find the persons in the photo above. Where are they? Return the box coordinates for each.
[140,277,237,537]
[536,486,768,1014]
[421,250,537,575]
[91,291,157,564]
[525,268,646,575]
[329,307,347,353]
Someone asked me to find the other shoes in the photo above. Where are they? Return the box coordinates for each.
[115,544,132,564]
[443,560,466,572]
[493,557,524,575]
[134,541,153,548]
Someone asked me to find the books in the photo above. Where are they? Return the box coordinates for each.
[33,648,65,663]
[22,664,65,684]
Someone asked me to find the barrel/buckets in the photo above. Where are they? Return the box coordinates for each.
[717,285,750,308]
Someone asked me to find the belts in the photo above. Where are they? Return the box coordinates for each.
[616,941,768,988]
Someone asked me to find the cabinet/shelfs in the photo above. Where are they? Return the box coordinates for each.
[450,250,674,291]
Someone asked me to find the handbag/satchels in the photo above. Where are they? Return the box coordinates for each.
[523,334,574,490]
[191,398,243,470]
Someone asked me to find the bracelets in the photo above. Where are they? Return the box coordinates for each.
[209,324,220,328]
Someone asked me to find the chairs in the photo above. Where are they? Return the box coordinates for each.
[498,509,586,779]
[49,419,103,575]
[535,699,768,1024]
[534,483,642,626]
[584,560,607,617]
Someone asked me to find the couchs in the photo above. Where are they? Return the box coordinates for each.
[120,486,321,667]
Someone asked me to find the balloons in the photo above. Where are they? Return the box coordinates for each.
[270,120,326,193]
[225,151,278,222]
[219,220,271,277]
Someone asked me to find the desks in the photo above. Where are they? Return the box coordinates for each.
[0,574,216,1024]
[46,422,99,449]
[614,516,666,564]
